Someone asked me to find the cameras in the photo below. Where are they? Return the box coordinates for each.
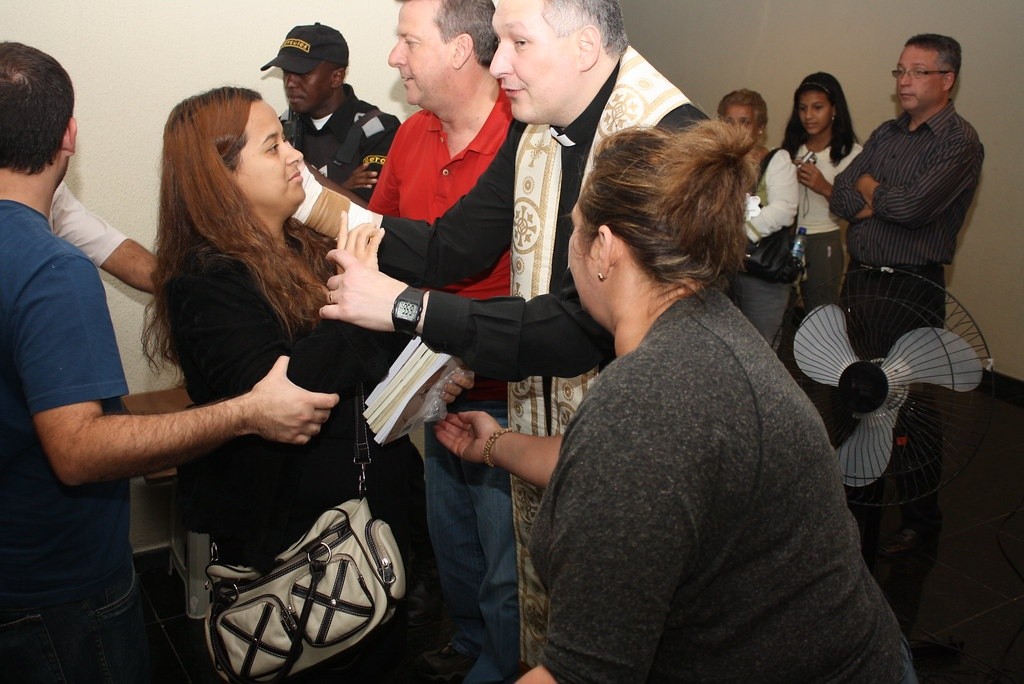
[797,151,817,169]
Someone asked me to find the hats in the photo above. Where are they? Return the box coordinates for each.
[259,21,348,72]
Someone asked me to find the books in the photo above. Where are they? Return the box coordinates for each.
[363,336,464,446]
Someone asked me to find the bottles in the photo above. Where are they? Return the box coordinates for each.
[791,228,806,261]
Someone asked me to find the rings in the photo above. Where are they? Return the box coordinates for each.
[328,291,334,303]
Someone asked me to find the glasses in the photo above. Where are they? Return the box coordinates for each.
[890,62,951,82]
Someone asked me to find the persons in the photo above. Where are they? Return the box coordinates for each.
[262,22,402,204]
[372,0,527,684]
[0,39,340,684]
[261,0,439,684]
[718,33,984,559]
[434,126,920,684]
[140,87,452,683]
[287,0,712,684]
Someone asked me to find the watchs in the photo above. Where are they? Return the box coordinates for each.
[392,286,429,337]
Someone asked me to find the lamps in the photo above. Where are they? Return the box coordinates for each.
[764,266,995,664]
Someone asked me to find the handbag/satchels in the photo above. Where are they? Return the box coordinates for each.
[741,148,796,282]
[192,494,407,684]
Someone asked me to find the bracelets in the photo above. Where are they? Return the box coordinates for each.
[482,427,513,468]
